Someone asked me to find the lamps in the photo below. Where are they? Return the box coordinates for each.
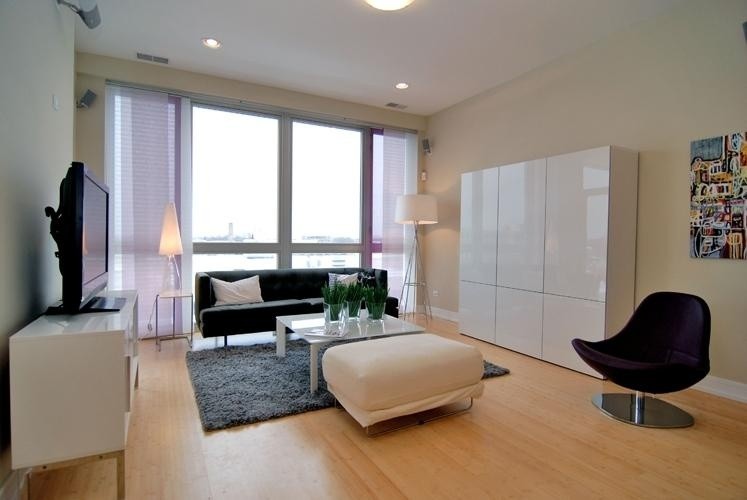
[158,200,184,293]
[393,194,439,320]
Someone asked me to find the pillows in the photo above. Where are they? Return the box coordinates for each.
[328,268,375,286]
[210,275,264,306]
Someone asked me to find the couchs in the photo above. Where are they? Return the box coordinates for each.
[195,268,398,347]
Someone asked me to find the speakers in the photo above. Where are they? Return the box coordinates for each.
[421,136,431,152]
[74,0,101,29]
[79,88,96,110]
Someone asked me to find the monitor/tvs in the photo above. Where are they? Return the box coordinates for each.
[41,159,128,317]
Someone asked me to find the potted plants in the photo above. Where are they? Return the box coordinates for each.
[321,282,390,333]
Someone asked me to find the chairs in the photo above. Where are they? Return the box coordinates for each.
[573,293,710,427]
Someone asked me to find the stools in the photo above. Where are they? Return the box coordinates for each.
[323,332,484,438]
[156,292,193,352]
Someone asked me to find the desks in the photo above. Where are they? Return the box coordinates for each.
[275,308,425,393]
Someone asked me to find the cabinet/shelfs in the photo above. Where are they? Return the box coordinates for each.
[544,146,639,382]
[497,159,546,360]
[8,288,138,499]
[459,168,498,344]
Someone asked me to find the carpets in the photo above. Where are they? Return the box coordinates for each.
[186,338,509,431]
